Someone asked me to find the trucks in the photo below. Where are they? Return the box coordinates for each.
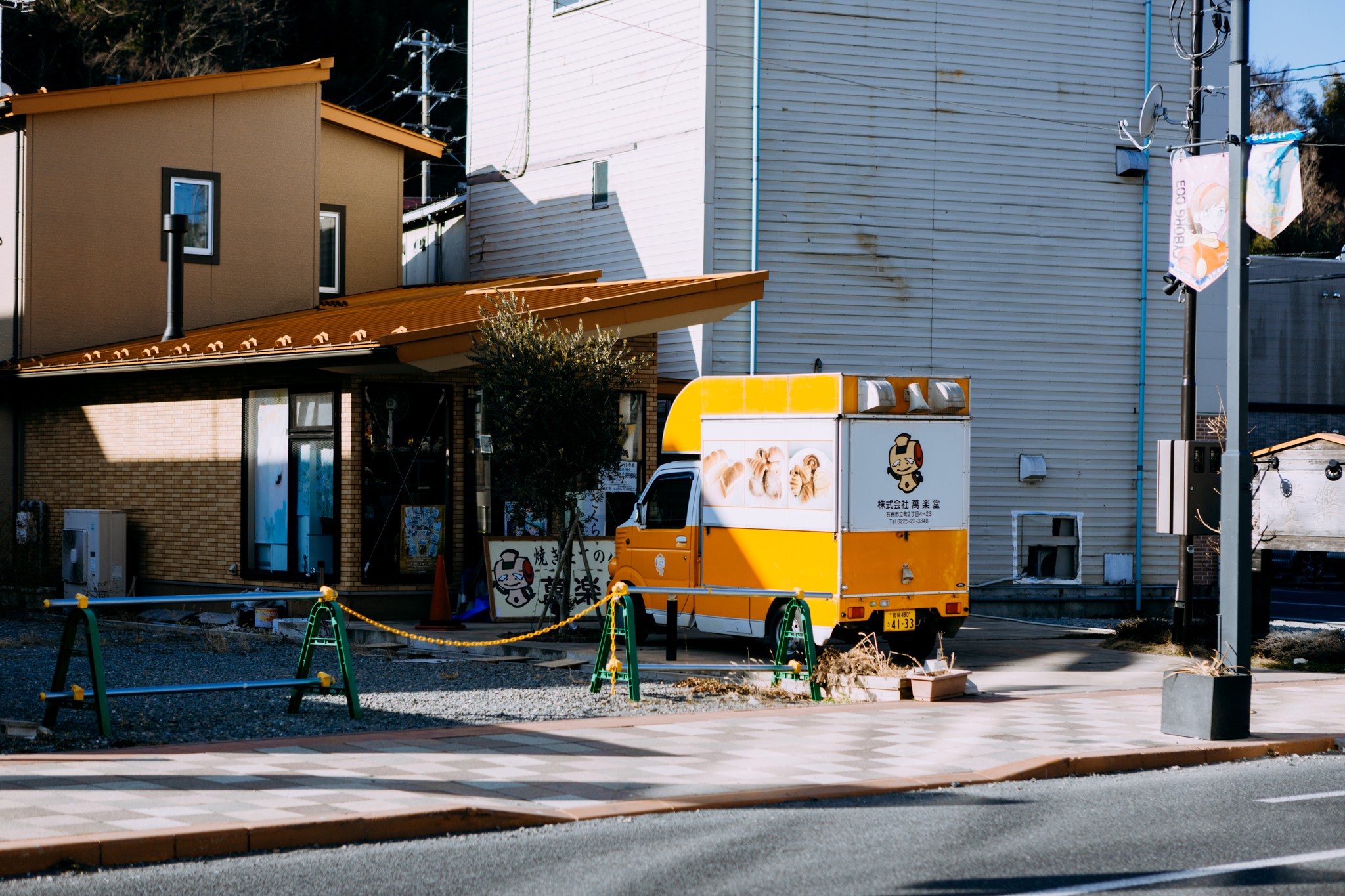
[605,372,970,665]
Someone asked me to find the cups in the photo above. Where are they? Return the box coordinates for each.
[317,560,325,591]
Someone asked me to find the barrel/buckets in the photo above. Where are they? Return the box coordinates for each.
[254,608,278,628]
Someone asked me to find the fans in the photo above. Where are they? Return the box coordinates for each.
[374,387,410,449]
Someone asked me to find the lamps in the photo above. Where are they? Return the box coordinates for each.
[1326,460,1345,480]
[1114,147,1149,177]
[1018,454,1048,483]
[1253,455,1279,475]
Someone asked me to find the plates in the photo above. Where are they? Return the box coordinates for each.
[789,448,833,505]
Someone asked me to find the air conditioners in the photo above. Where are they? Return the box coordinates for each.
[61,508,129,600]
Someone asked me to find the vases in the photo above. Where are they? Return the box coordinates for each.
[906,669,972,703]
[821,672,911,700]
